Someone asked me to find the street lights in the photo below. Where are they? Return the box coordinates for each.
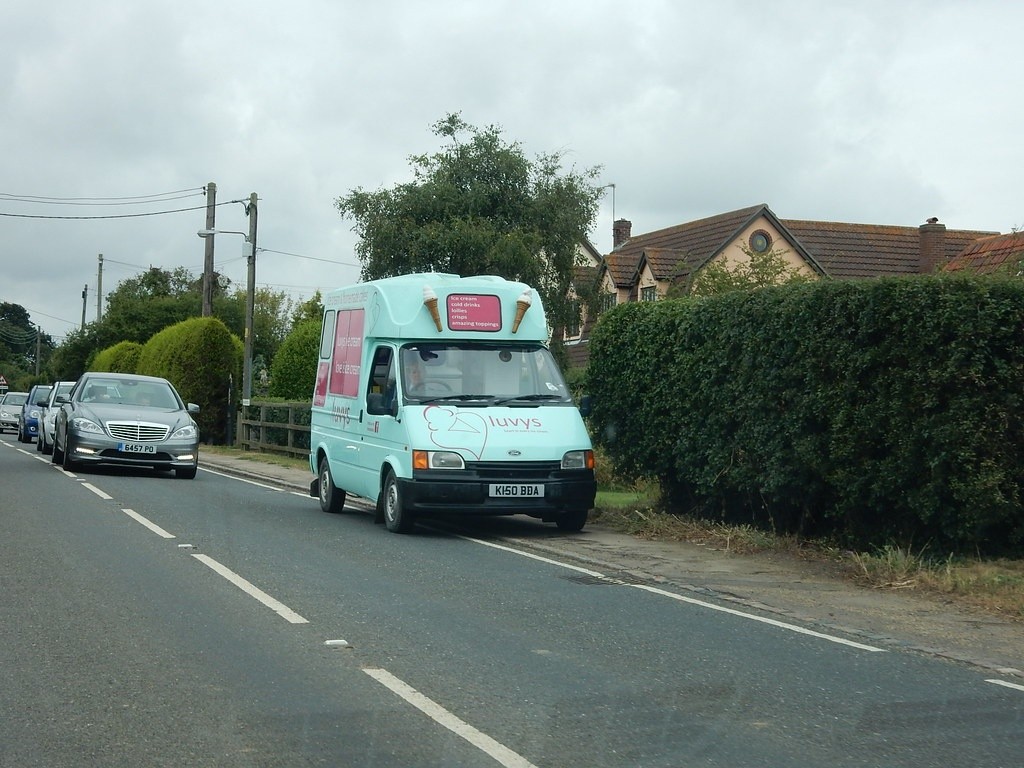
[197,227,258,451]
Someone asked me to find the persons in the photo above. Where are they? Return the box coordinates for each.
[408,362,434,391]
[83,385,110,402]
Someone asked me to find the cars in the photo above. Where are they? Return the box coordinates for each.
[0,391,29,433]
[37,381,77,454]
[18,384,53,443]
[52,372,200,479]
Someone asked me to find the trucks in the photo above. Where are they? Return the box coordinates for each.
[309,272,598,533]
